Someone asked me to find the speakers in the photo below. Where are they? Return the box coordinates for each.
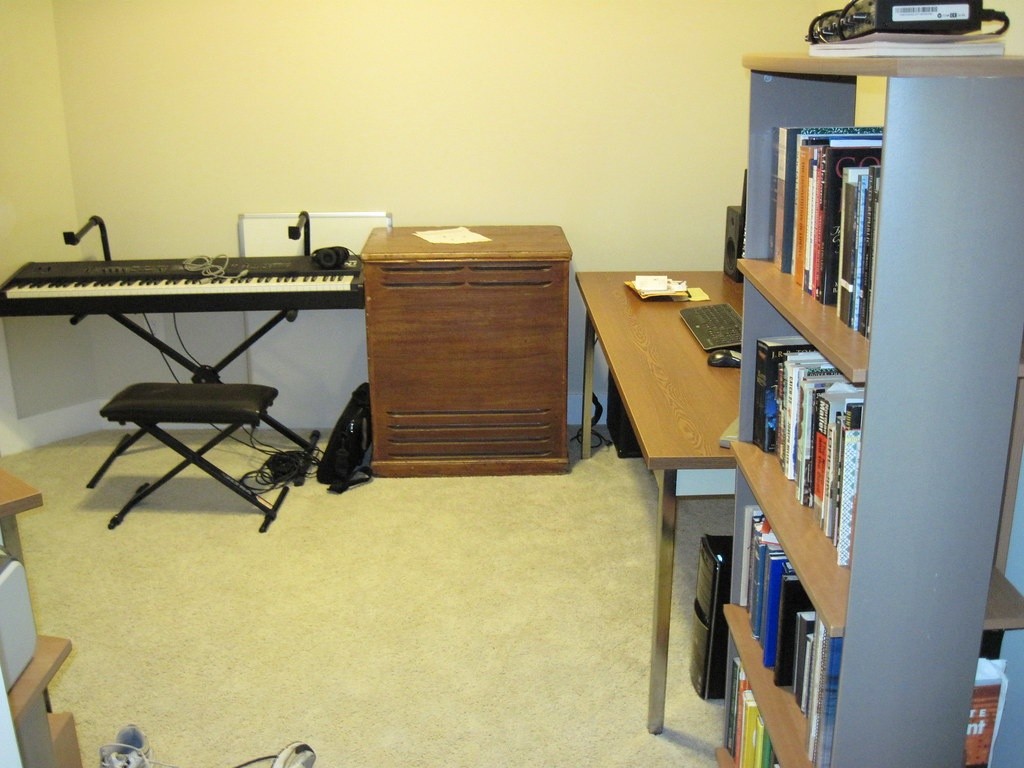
[724,205,744,283]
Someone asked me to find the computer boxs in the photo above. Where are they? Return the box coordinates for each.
[688,534,734,700]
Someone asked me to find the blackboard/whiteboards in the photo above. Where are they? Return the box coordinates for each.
[237,211,393,431]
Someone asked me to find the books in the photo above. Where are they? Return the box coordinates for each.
[751,335,865,568]
[806,32,1006,59]
[725,504,845,768]
[624,274,693,299]
[766,122,882,342]
[964,654,1010,767]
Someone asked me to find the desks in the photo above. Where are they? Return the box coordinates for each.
[575,271,744,734]
[0,471,52,715]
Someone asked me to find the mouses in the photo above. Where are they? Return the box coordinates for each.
[707,350,741,368]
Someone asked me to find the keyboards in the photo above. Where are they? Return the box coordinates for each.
[679,303,743,351]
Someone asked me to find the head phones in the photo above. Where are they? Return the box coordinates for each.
[311,245,350,268]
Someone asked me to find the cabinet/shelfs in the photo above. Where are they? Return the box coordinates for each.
[715,54,1024,768]
[8,634,82,768]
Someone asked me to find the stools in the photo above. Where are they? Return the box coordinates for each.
[100,382,289,533]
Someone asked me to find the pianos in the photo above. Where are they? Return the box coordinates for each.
[0,255,364,315]
